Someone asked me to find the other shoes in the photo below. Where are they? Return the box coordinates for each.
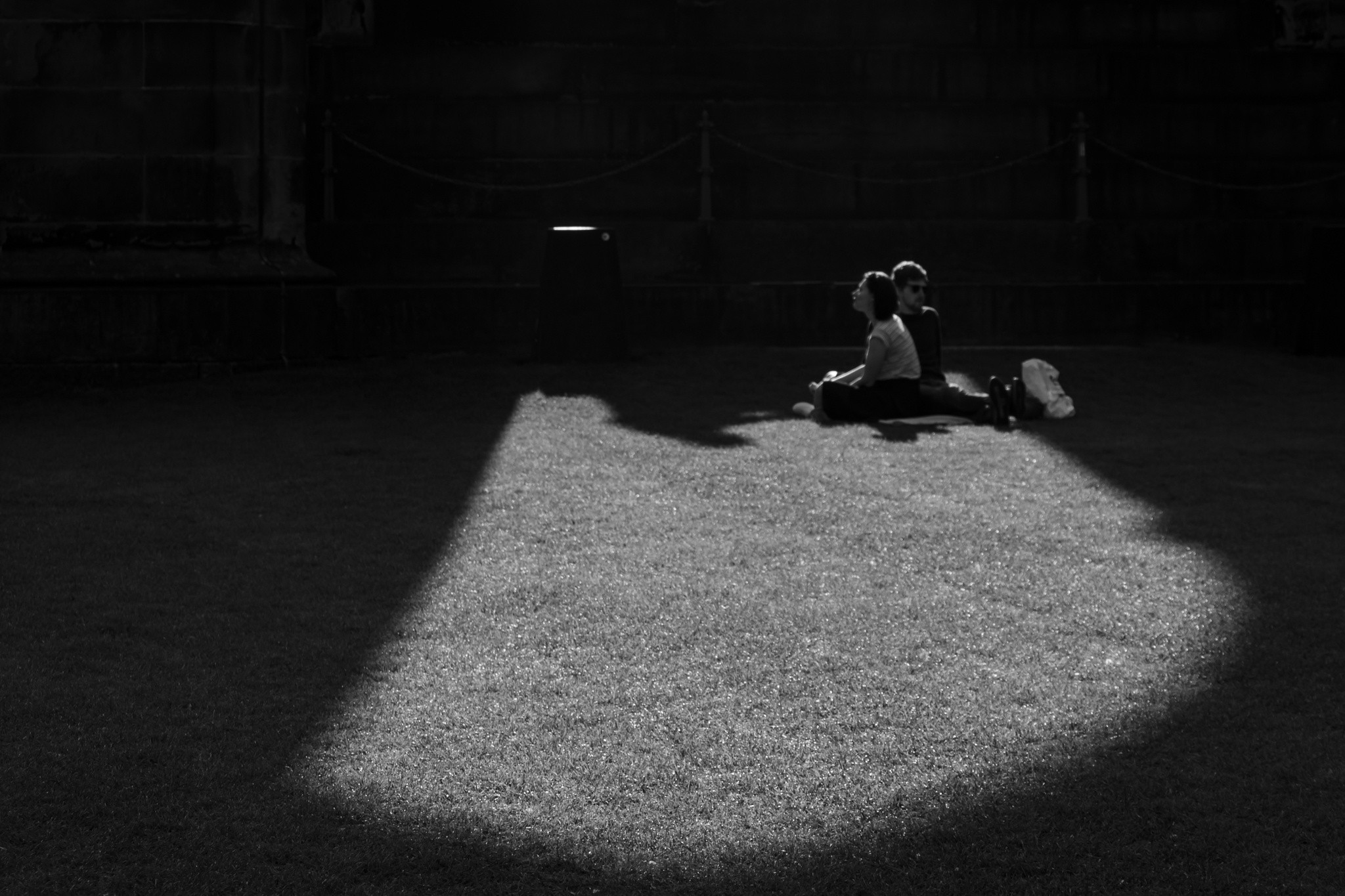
[1008,377,1027,417]
[792,402,814,418]
[989,377,1012,429]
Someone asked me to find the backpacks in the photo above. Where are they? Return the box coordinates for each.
[1021,359,1074,418]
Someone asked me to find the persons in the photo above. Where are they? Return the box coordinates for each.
[792,272,920,421]
[892,261,1026,427]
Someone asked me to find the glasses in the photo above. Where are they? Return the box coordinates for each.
[907,283,927,293]
